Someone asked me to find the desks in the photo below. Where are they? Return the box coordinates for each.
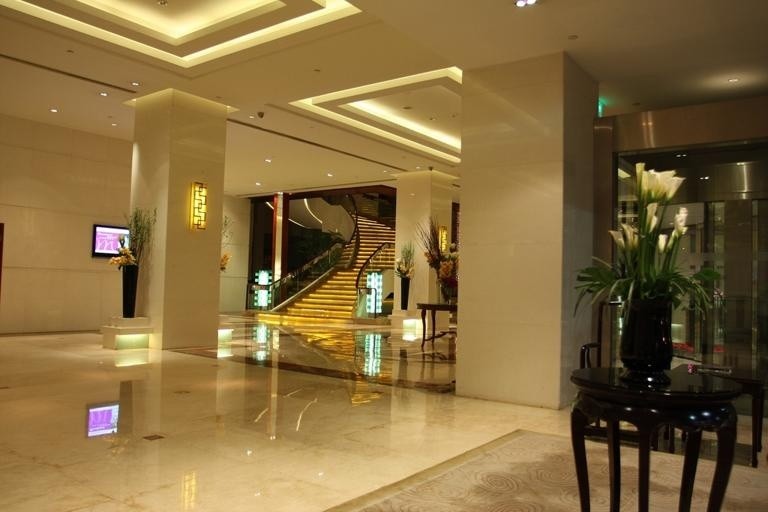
[570,369,745,511]
[414,302,457,348]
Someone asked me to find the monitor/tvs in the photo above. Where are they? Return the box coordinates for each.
[91,224,130,257]
[85,401,120,439]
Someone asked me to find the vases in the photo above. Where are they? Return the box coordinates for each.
[620,285,674,386]
[122,263,137,318]
[402,277,410,309]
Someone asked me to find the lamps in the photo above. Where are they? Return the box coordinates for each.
[188,180,210,232]
[254,269,275,310]
[437,223,448,255]
[366,270,383,318]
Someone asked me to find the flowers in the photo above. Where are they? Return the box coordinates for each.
[393,241,413,280]
[414,215,457,289]
[107,206,157,269]
[568,160,729,325]
